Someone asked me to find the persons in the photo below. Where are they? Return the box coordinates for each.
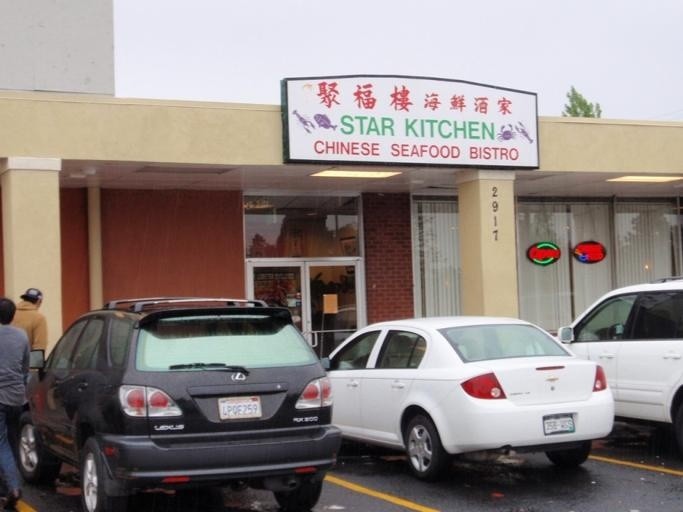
[7,286,49,372]
[0,297,31,512]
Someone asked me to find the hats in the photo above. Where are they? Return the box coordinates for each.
[20,288,43,304]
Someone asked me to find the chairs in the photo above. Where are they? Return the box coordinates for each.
[368,331,542,368]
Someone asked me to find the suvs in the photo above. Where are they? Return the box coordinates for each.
[13,296,344,511]
[518,289,593,337]
[554,276,682,458]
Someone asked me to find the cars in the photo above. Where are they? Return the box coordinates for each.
[313,298,356,356]
[321,314,617,485]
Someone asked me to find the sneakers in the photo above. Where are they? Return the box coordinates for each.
[5,488,21,510]
